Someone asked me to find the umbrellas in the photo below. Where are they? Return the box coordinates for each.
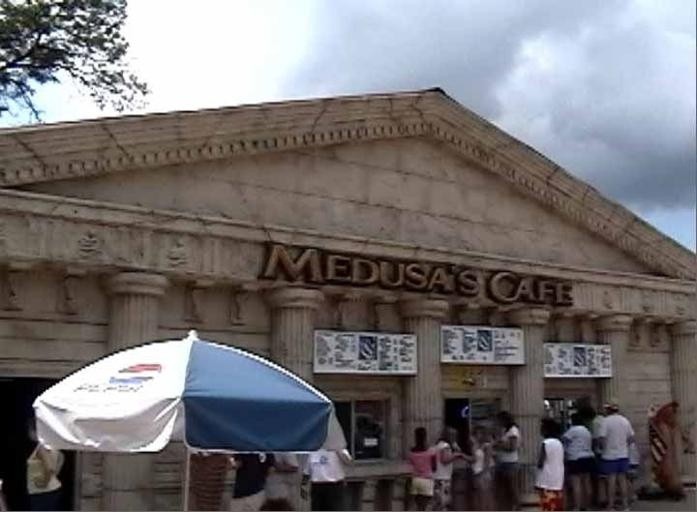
[32,328,347,512]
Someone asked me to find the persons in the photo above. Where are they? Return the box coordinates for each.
[407,400,642,512]
[260,497,295,511]
[27,417,65,511]
[232,453,269,512]
[300,448,354,511]
